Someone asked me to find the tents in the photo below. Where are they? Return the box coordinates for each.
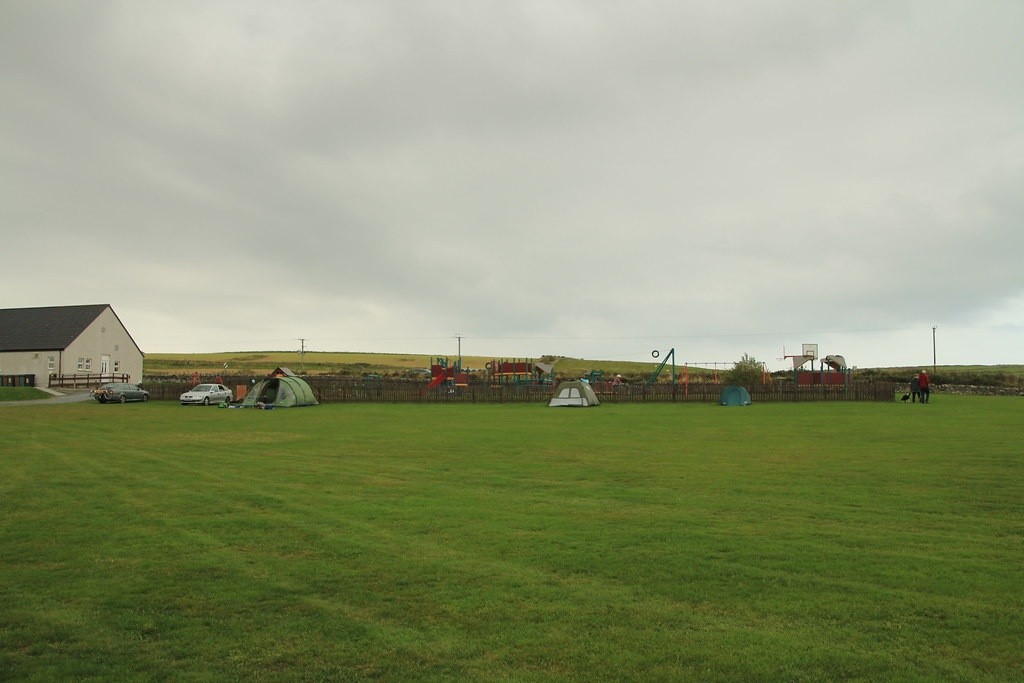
[720,385,751,406]
[235,376,319,407]
[549,381,600,407]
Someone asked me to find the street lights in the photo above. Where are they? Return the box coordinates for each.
[932,325,937,373]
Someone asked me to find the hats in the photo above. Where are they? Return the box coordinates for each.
[914,374,919,379]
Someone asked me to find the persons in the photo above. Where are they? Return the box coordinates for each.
[614,375,622,384]
[911,370,930,403]
[192,370,223,384]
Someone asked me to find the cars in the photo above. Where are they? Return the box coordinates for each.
[179,384,234,406]
[91,383,151,404]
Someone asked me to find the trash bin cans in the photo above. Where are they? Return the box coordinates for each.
[2,375,8,386]
[8,375,14,387]
[24,374,35,387]
[13,375,19,387]
[19,375,25,386]
[0,375,3,386]
